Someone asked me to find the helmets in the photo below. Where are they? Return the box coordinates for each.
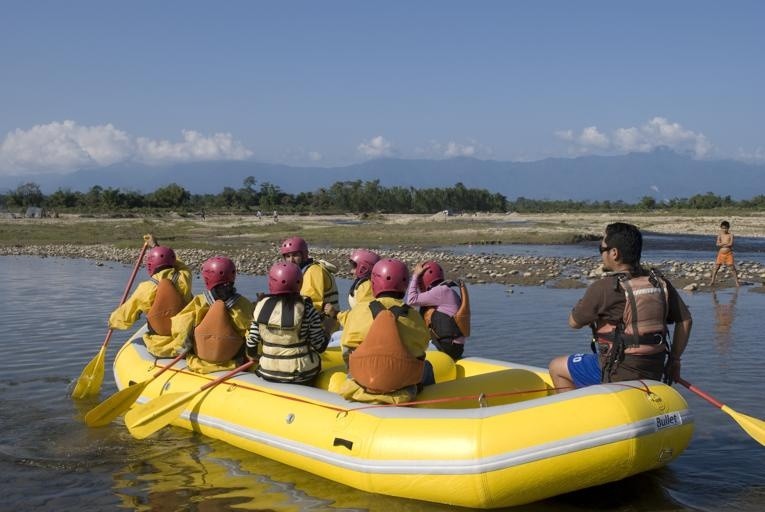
[371,258,410,298]
[348,249,381,280]
[268,261,303,294]
[416,261,445,294]
[280,237,308,262]
[146,247,176,276]
[201,257,237,290]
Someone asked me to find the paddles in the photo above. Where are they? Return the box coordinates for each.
[676,377,765,446]
[85,346,195,427]
[73,242,148,398]
[125,356,261,440]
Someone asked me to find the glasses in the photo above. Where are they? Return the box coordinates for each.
[599,244,609,255]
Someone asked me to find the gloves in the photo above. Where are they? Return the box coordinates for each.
[143,233,157,247]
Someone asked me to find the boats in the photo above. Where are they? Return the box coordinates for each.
[112,323,694,507]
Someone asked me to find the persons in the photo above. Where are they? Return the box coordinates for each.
[280,237,340,334]
[200,208,206,221]
[341,258,436,395]
[256,210,262,221]
[171,257,262,375]
[325,249,381,327]
[711,288,739,350]
[107,233,195,359]
[408,258,465,363]
[707,221,740,287]
[246,262,331,387]
[548,223,693,394]
[272,209,279,222]
